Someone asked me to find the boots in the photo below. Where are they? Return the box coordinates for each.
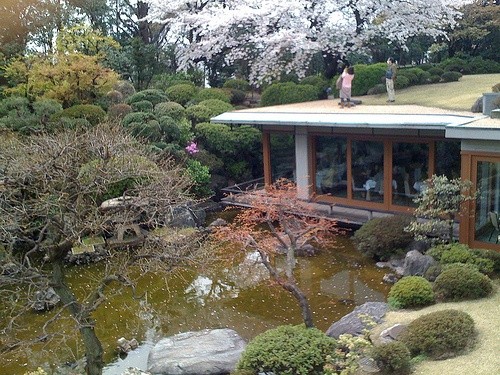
[347,102,350,107]
[339,102,344,109]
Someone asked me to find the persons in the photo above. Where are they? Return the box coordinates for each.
[315,137,461,209]
[386,57,397,102]
[340,65,356,109]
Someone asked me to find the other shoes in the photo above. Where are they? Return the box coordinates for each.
[387,99,395,103]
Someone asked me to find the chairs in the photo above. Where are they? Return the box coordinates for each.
[403,181,418,207]
[487,211,500,245]
[351,175,367,199]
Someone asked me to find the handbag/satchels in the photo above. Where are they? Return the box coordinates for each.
[336,74,343,89]
